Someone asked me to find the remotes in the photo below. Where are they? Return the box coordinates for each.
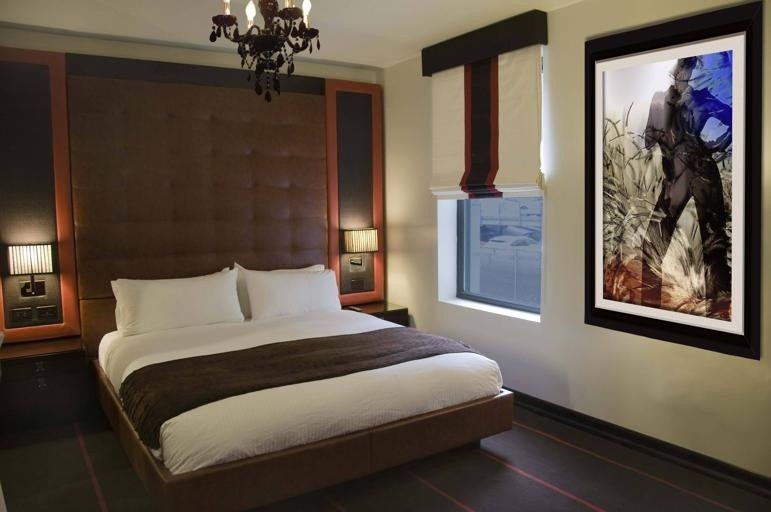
[350,306,362,312]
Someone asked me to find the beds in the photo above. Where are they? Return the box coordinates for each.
[92,322,514,512]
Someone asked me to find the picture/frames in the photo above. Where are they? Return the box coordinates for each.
[584,0,764,361]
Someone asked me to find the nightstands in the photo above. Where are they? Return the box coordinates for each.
[0,336,86,448]
[341,300,410,327]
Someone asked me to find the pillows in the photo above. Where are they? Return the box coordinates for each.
[110,266,239,338]
[233,262,343,324]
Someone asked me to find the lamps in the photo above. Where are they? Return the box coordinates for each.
[210,1,320,105]
[7,244,54,293]
[342,228,378,254]
